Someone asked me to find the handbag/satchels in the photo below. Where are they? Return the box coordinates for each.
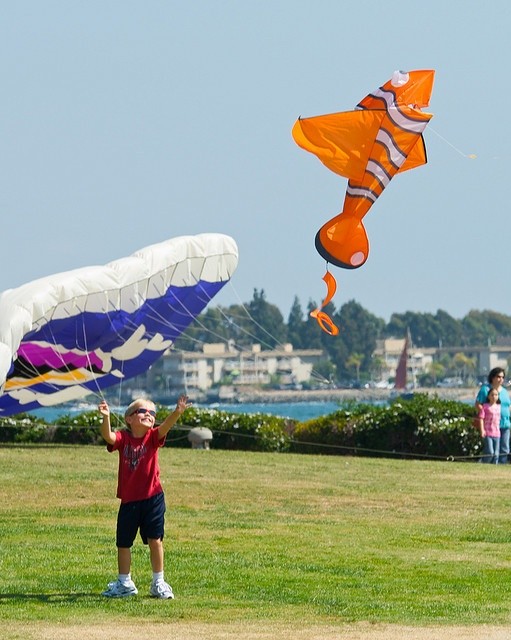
[472,414,480,432]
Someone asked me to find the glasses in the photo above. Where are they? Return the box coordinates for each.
[496,376,505,379]
[129,408,156,417]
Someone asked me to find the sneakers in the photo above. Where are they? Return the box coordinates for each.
[150,578,174,599]
[101,579,138,598]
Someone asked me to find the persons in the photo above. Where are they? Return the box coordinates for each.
[95,393,189,600]
[475,387,502,465]
[475,367,510,465]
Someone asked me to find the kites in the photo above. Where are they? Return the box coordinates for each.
[0,232,238,417]
[293,70,435,336]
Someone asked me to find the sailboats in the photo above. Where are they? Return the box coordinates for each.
[387,326,428,404]
